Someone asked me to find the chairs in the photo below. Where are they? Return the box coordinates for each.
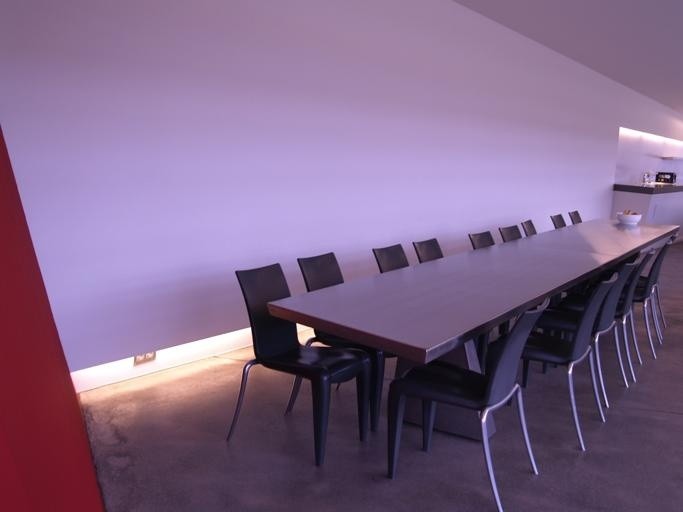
[373,244,410,273]
[521,271,621,454]
[284,252,395,433]
[521,220,537,236]
[226,263,372,464]
[413,238,444,264]
[615,233,676,384]
[550,215,566,228]
[549,261,638,391]
[499,225,522,242]
[568,211,582,224]
[384,298,552,511]
[468,231,495,249]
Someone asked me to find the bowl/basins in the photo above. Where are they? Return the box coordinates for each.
[617,213,641,227]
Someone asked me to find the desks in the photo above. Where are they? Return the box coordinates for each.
[265,215,680,451]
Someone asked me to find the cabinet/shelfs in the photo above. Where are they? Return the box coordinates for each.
[614,184,683,243]
